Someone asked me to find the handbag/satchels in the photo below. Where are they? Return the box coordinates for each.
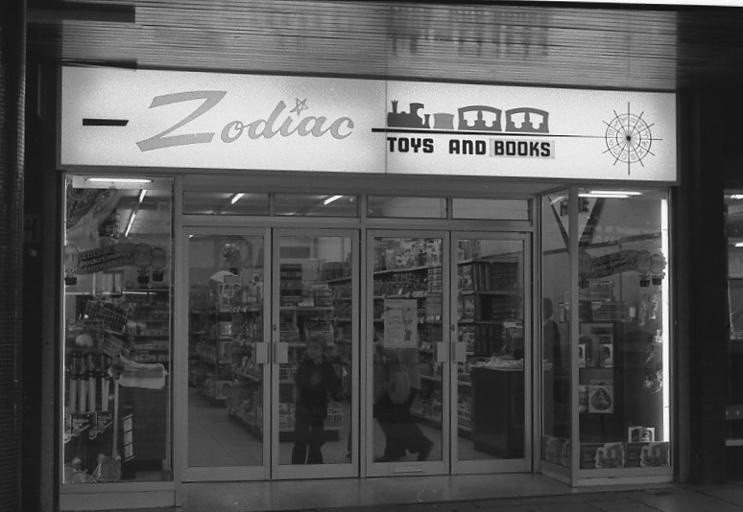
[387,371,410,403]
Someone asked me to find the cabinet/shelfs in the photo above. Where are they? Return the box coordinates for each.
[69,261,625,474]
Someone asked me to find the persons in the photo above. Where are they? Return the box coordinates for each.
[284,333,350,464]
[374,329,433,460]
[513,296,562,432]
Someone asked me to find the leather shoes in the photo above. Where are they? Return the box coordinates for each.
[416,441,433,461]
